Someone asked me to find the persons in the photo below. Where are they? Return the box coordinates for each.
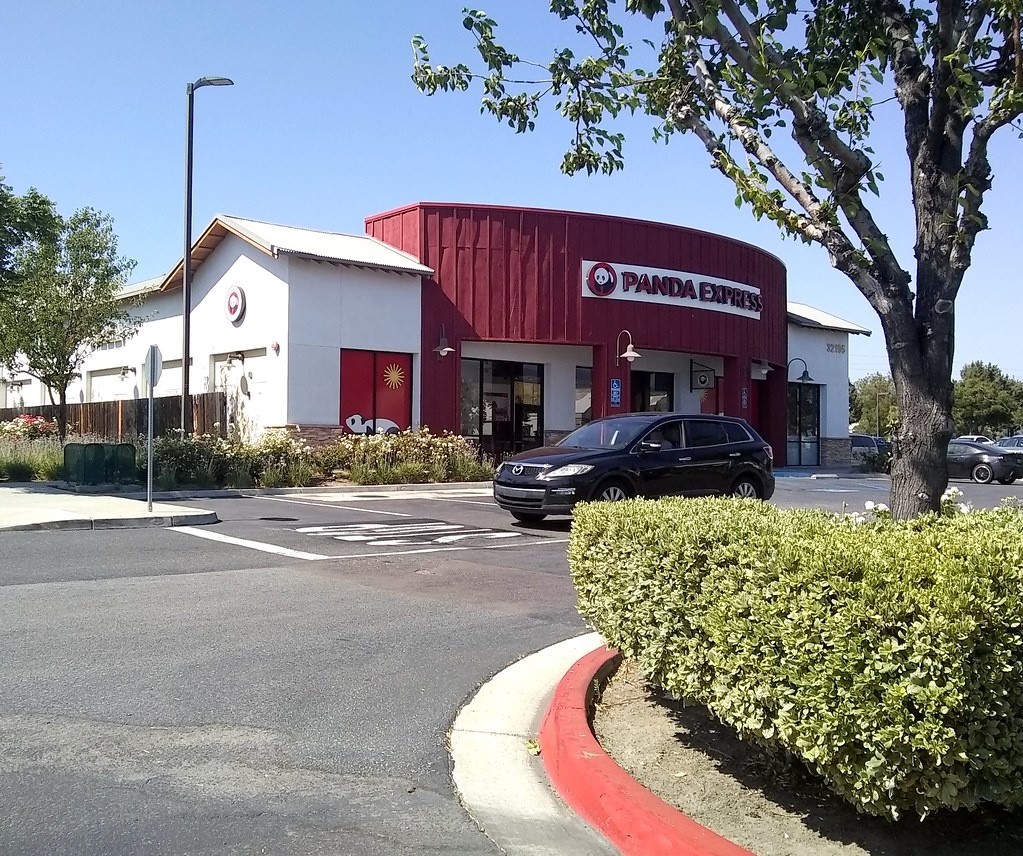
[647,426,673,450]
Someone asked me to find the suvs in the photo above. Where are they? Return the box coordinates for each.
[492,411,776,524]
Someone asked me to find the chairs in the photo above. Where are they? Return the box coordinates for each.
[643,430,673,450]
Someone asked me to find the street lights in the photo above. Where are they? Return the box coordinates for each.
[876,392,891,437]
[180,75,236,441]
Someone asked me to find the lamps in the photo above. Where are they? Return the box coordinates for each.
[755,358,774,376]
[221,353,247,369]
[6,382,23,393]
[431,323,455,359]
[616,330,642,367]
[787,358,815,385]
[117,366,136,382]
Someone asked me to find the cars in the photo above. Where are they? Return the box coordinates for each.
[954,435,1023,452]
[946,439,1023,484]
[848,434,890,466]
